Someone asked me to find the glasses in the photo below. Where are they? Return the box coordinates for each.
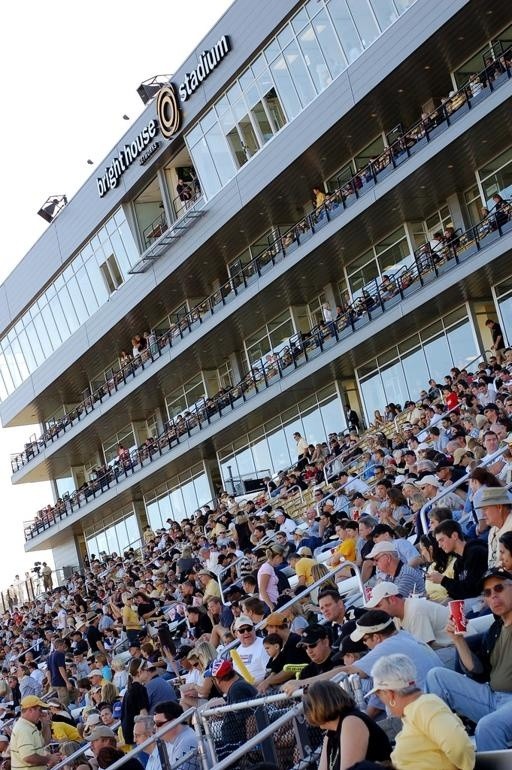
[238,626,252,634]
[484,584,503,597]
[151,720,168,727]
[302,642,317,649]
[127,597,133,600]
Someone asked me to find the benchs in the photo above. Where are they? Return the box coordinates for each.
[253,411,407,518]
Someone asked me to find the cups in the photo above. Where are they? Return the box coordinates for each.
[353,510,358,520]
[448,600,467,635]
[365,587,372,601]
[49,742,60,754]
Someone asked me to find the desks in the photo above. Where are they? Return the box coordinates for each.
[146,224,167,237]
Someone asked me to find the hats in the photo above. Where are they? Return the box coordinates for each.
[363,676,415,699]
[271,512,284,519]
[289,528,304,536]
[365,541,397,558]
[391,448,467,487]
[478,567,511,581]
[84,669,122,741]
[204,659,233,677]
[259,612,288,630]
[473,487,512,509]
[364,582,400,608]
[20,695,51,709]
[224,585,242,594]
[350,617,393,643]
[235,616,253,628]
[296,624,326,648]
[197,569,213,577]
[479,403,498,409]
[61,612,102,640]
[347,489,364,501]
[296,546,313,556]
[366,524,393,540]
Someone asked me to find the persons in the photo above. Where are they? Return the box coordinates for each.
[0,53,512,770]
